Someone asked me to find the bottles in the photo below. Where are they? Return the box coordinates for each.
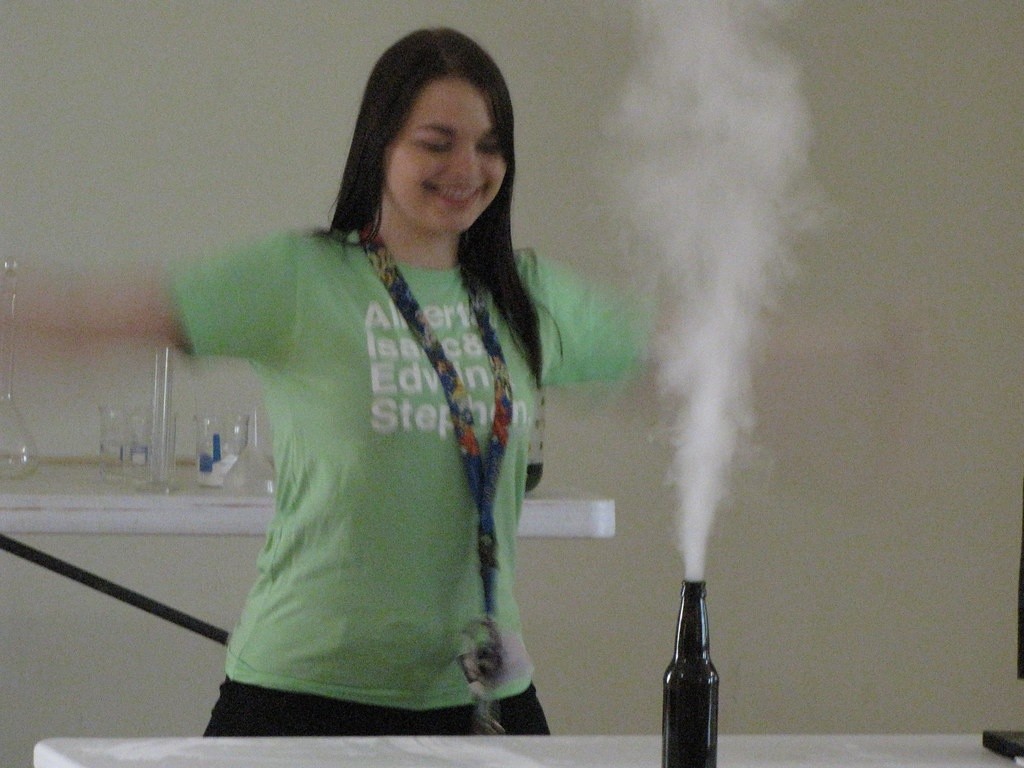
[661,579,718,768]
[0,260,39,478]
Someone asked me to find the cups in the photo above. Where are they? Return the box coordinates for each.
[96,404,176,486]
[193,412,251,486]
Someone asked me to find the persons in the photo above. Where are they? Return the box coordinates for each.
[0,26,667,737]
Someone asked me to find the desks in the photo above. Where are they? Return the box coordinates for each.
[33,733,1016,768]
